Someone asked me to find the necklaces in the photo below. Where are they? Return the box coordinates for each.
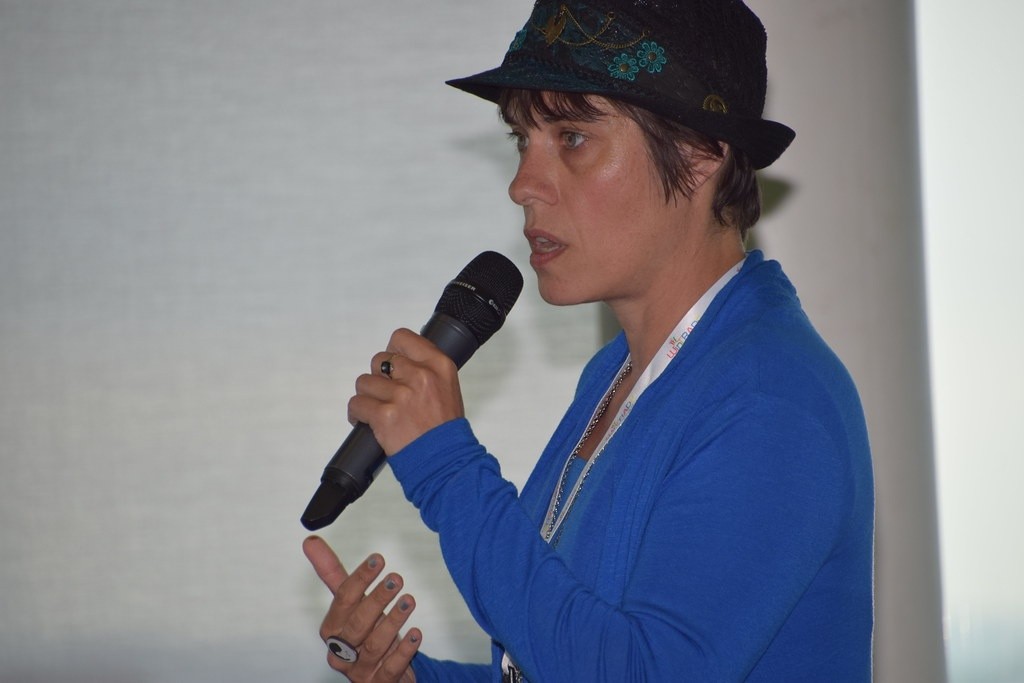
[516,362,632,683]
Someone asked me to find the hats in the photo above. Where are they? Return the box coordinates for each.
[442,0,796,171]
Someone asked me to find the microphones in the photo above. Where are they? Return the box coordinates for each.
[301,251,524,532]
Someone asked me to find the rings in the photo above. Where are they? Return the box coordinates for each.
[325,636,359,663]
[380,354,404,379]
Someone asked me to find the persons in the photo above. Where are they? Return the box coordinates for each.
[302,0,875,682]
